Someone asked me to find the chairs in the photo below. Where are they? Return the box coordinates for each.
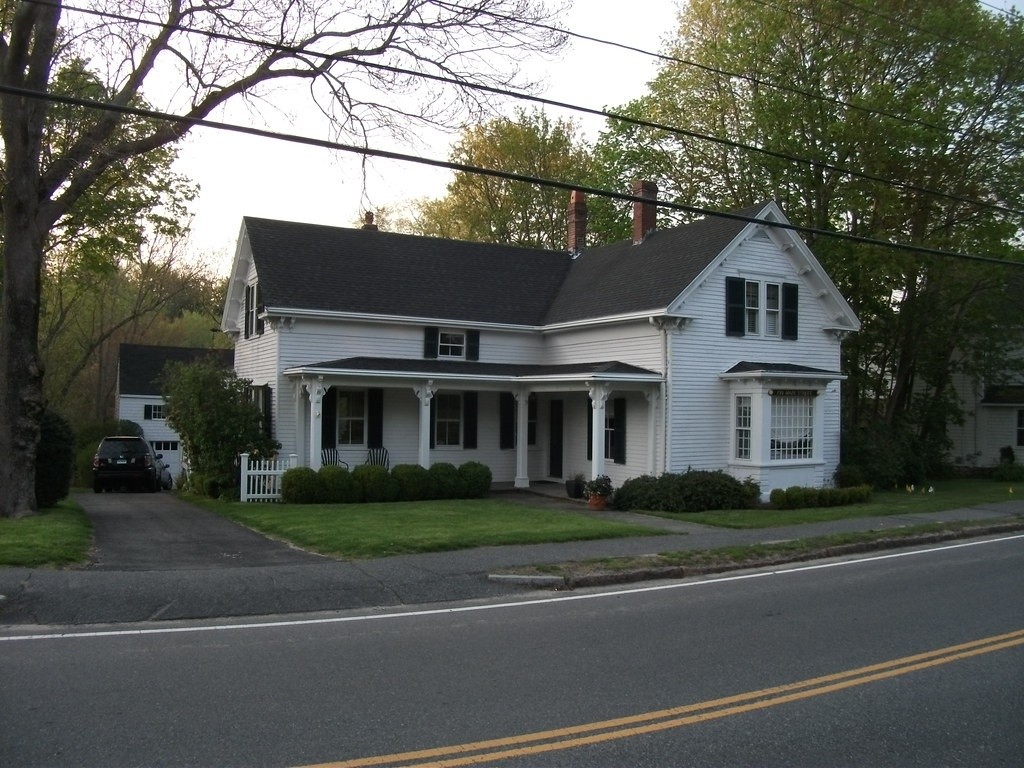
[321,447,349,470]
[365,446,390,471]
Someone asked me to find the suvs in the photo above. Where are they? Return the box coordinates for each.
[93,435,173,493]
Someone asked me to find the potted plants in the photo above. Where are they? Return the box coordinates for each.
[560,472,587,499]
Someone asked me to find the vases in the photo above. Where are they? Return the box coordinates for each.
[587,495,608,511]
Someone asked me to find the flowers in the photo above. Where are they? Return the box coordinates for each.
[585,474,614,497]
